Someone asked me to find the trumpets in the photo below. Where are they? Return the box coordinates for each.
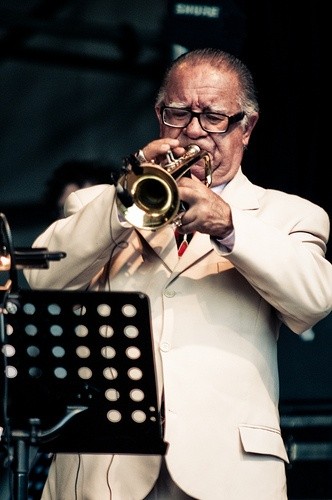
[113,143,212,231]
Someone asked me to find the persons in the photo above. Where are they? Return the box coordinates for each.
[24,47,332,500]
[45,159,112,220]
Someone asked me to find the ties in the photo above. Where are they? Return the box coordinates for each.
[173,201,195,258]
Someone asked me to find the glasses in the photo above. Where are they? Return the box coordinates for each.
[160,101,246,133]
